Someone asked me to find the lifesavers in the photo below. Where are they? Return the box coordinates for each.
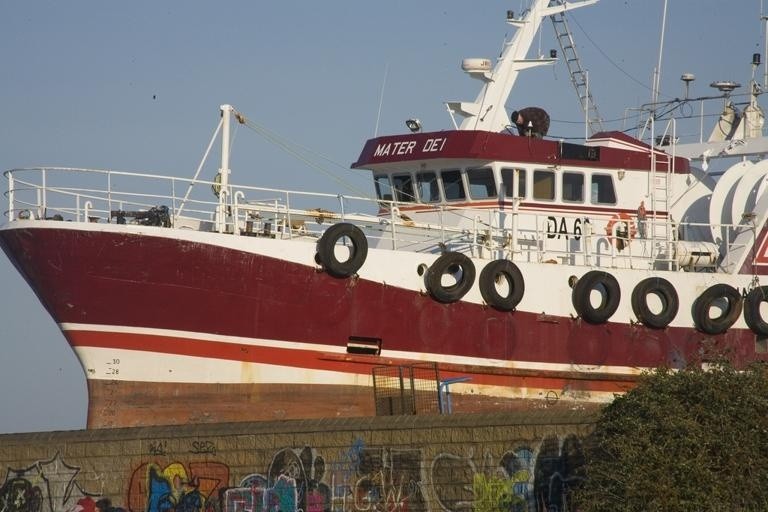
[607,212,637,249]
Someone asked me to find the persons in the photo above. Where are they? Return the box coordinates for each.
[511,107,551,138]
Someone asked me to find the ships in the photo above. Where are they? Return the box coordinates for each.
[0,0,768,431]
